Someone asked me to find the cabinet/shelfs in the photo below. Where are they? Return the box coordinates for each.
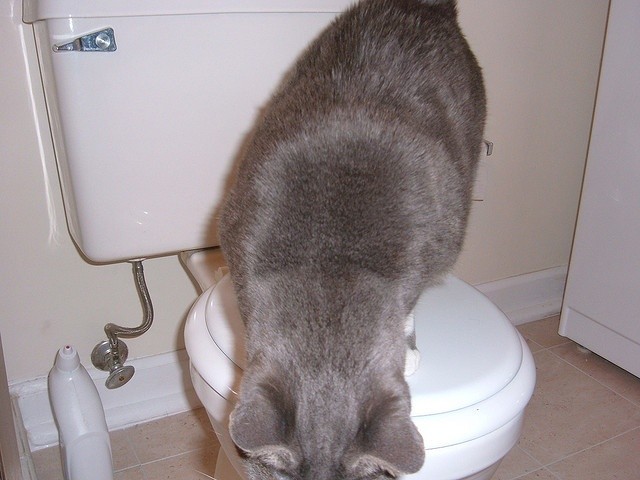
[558,0,640,380]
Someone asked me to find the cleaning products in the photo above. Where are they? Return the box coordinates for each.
[48,346,116,480]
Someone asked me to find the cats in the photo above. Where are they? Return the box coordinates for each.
[215,0,488,480]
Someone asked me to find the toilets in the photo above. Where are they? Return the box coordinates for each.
[22,1,537,479]
